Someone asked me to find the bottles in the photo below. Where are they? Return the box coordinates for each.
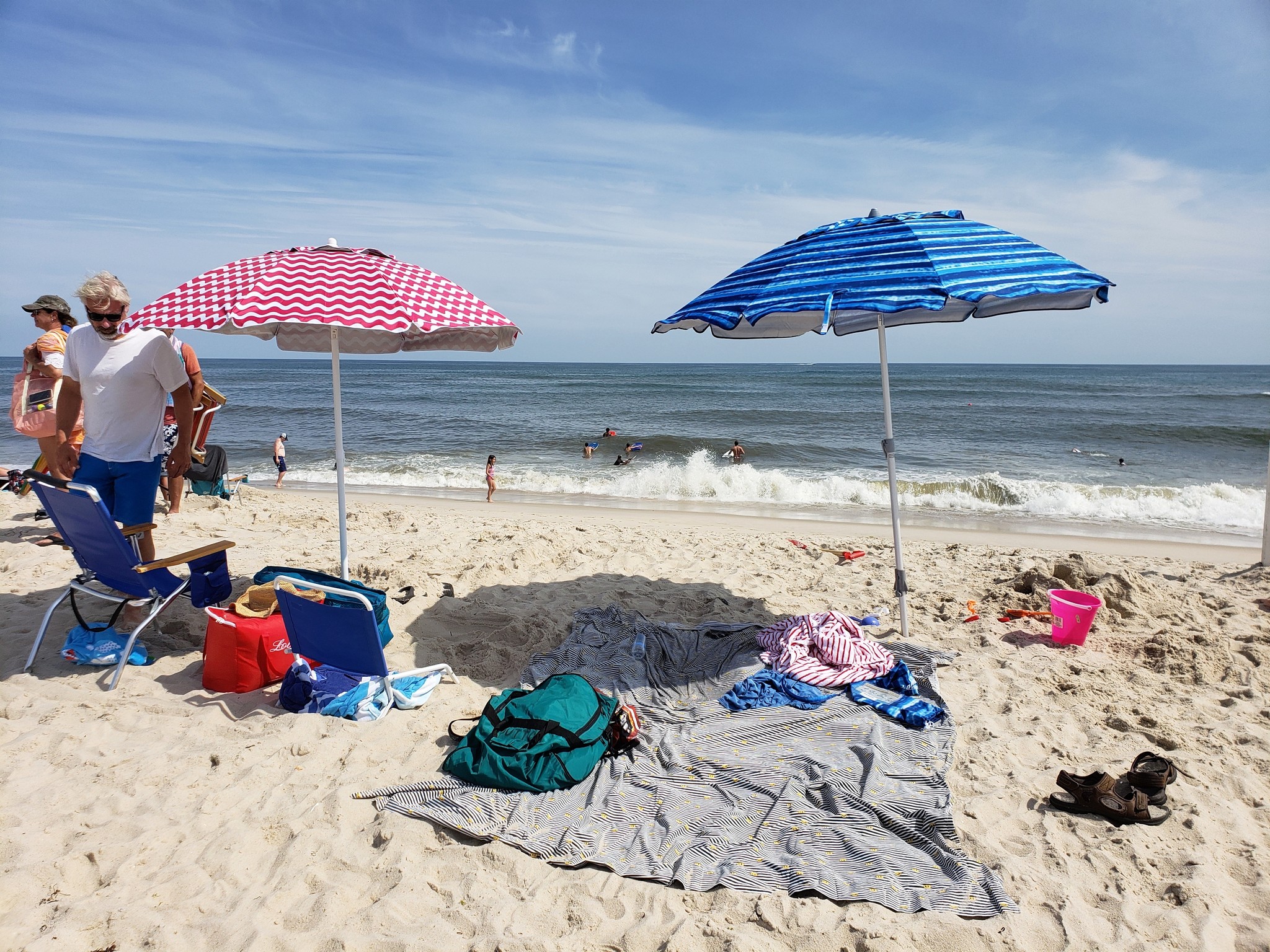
[632,634,646,659]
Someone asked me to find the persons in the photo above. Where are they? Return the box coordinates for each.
[1118,458,1126,466]
[728,441,745,457]
[158,328,204,513]
[603,428,612,437]
[626,443,642,452]
[613,455,629,465]
[56,273,194,630]
[486,455,496,503]
[273,433,289,487]
[10,295,85,547]
[584,442,594,454]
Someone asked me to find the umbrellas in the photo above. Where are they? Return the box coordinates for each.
[116,239,523,580]
[650,208,1117,636]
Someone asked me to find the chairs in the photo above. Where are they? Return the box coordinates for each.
[22,469,235,691]
[184,444,249,505]
[274,576,460,702]
[190,380,228,464]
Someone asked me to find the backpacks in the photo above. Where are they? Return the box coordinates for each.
[442,673,620,791]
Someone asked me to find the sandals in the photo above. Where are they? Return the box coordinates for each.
[1127,752,1196,805]
[1049,769,1169,825]
[35,509,50,521]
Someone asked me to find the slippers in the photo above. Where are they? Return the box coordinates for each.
[36,536,66,546]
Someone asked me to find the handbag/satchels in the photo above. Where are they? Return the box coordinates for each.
[202,589,324,694]
[253,566,393,649]
[9,331,84,437]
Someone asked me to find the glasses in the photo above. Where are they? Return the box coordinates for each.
[34,309,50,316]
[85,304,125,321]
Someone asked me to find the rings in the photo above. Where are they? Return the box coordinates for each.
[184,468,188,469]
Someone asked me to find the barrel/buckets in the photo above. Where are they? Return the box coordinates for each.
[1047,589,1103,646]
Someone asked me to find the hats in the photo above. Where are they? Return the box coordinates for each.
[21,295,71,316]
[229,580,326,618]
[280,433,288,441]
